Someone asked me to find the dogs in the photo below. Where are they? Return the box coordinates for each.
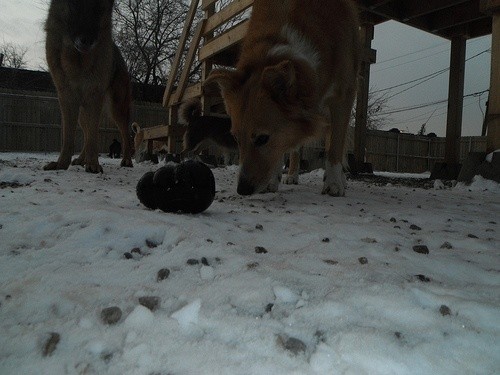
[43,1,150,173]
[180,99,238,161]
[199,0,359,197]
